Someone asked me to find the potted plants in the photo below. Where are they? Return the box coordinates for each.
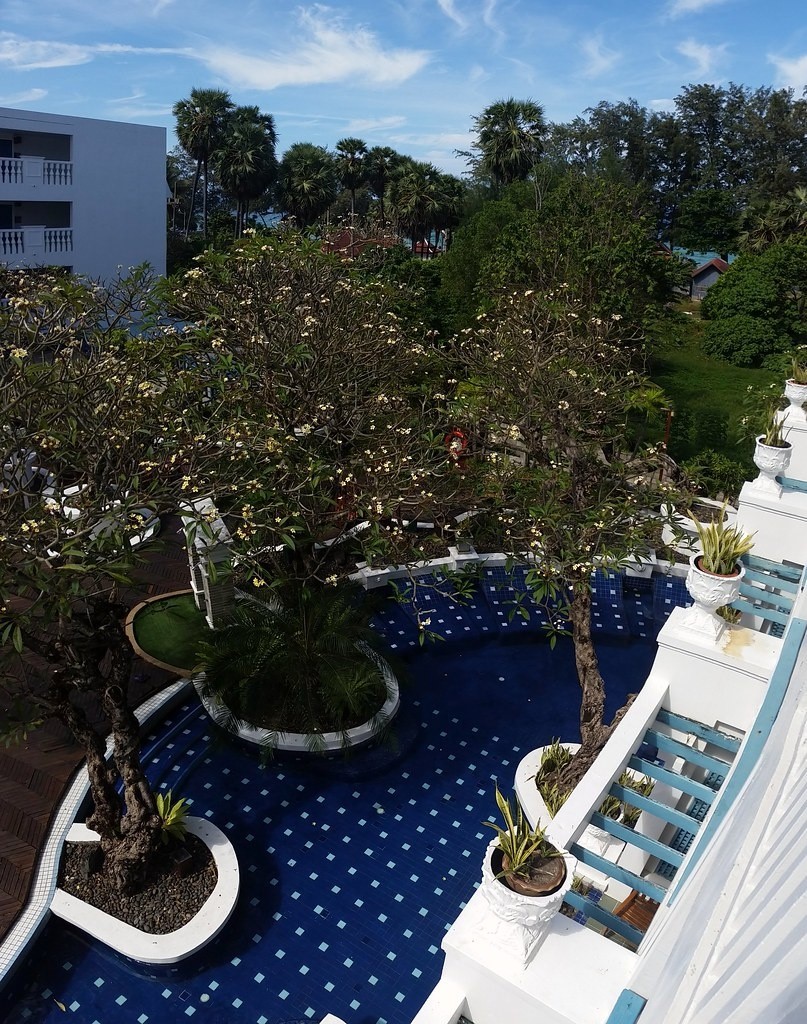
[481,781,575,924]
[634,531,656,554]
[675,496,756,643]
[776,356,807,430]
[455,516,476,553]
[751,403,794,499]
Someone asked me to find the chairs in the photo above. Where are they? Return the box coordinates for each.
[602,890,661,950]
